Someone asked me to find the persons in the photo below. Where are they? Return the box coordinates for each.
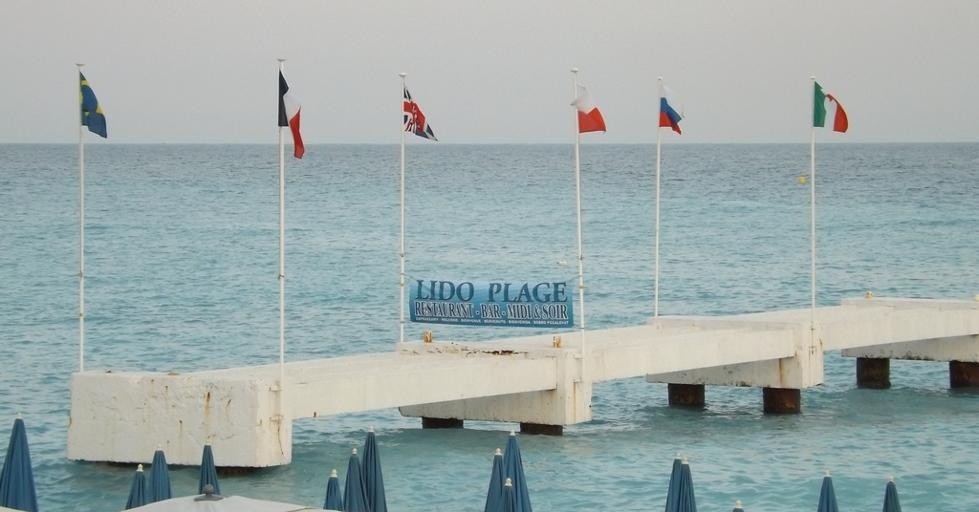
[732,498,744,511]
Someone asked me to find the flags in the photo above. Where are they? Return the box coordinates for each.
[659,83,686,136]
[278,69,304,160]
[571,72,607,135]
[403,86,438,142]
[78,70,107,140]
[813,80,849,135]
